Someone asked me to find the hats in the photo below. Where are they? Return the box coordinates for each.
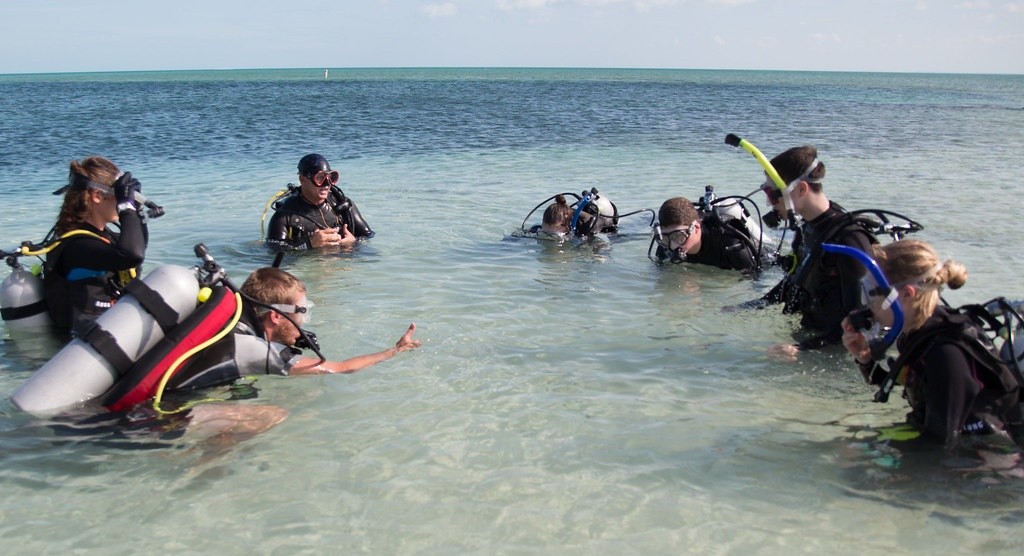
[298,154,335,186]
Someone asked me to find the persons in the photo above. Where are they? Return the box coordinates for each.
[530,195,617,239]
[267,154,372,250]
[44,157,150,344]
[841,240,1024,444]
[763,146,876,349]
[658,197,757,270]
[168,267,421,389]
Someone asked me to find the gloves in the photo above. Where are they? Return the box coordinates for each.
[113,171,142,208]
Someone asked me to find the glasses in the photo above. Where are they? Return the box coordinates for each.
[763,186,783,205]
[314,171,339,184]
[655,224,694,246]
[536,230,566,240]
[859,277,881,305]
[300,301,314,324]
